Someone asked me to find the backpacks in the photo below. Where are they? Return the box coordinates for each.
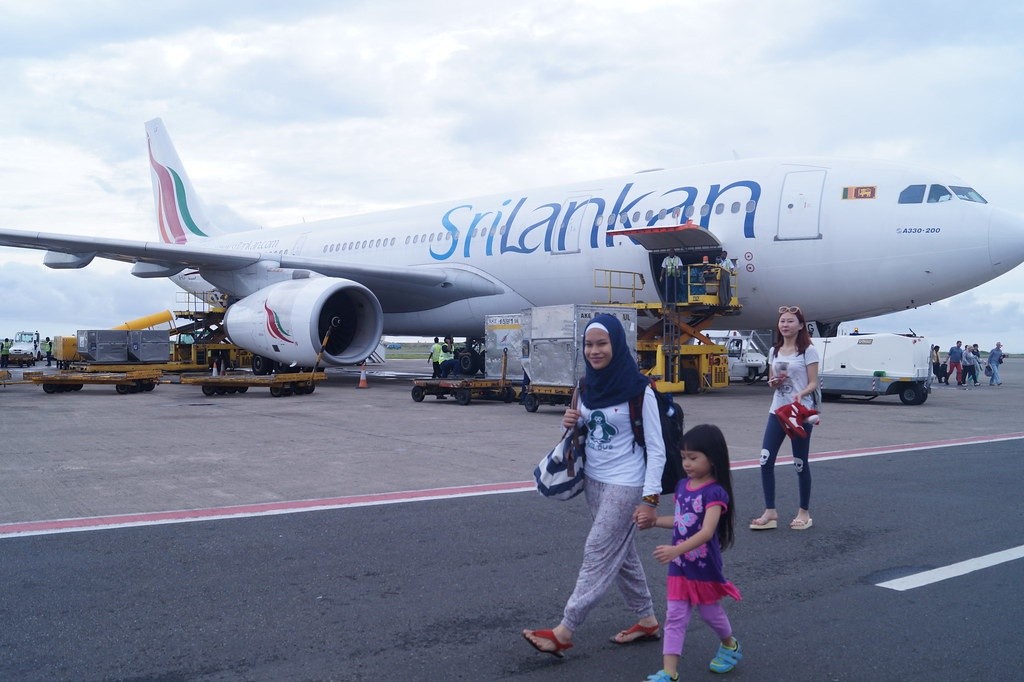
[630,376,685,495]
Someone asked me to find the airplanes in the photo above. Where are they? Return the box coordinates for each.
[0,116,1024,379]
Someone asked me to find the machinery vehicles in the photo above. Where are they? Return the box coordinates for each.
[481,304,638,413]
[0,290,340,396]
[812,329,934,405]
[589,263,771,399]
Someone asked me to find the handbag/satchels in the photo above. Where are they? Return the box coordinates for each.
[985,365,993,376]
[533,376,586,501]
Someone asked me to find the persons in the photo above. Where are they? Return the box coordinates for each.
[632,424,744,682]
[933,341,1006,386]
[659,248,684,301]
[427,336,460,399]
[522,313,667,658]
[750,307,820,529]
[45,337,53,366]
[716,251,736,307]
[0,338,11,368]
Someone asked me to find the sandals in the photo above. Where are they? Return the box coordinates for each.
[522,629,575,658]
[610,623,661,644]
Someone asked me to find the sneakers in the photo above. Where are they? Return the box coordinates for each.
[710,637,742,673]
[773,401,821,441]
[644,670,679,682]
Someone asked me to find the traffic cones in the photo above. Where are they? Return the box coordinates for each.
[219,359,226,377]
[212,362,219,377]
[356,362,370,389]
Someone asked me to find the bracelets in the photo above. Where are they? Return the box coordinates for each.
[642,501,656,508]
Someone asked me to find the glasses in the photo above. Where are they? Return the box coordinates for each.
[779,306,801,314]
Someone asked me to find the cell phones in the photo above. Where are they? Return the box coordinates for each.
[767,378,782,383]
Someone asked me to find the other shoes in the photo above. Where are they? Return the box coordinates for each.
[958,381,962,385]
[437,395,447,399]
[974,383,980,386]
[944,379,949,384]
[962,384,968,386]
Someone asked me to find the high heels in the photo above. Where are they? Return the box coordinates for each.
[749,517,778,528]
[791,518,812,529]
